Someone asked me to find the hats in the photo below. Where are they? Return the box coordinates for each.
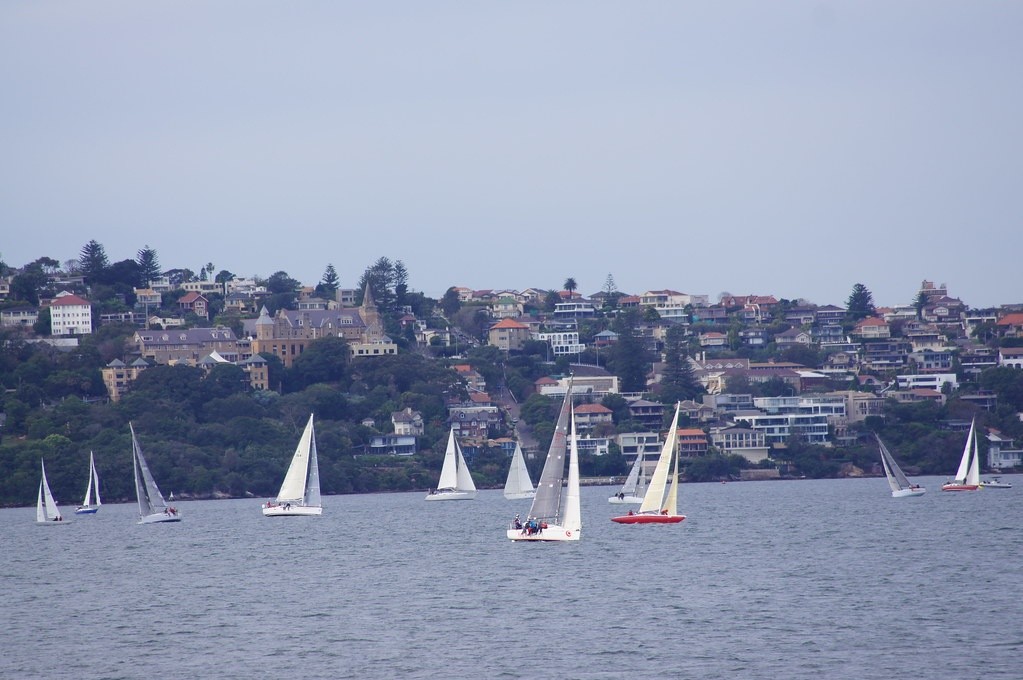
[534,517,537,521]
[516,515,519,518]
[528,515,533,519]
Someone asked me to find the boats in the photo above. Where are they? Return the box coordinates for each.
[980,475,1013,489]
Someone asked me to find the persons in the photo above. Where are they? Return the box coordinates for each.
[431,489,444,495]
[981,479,1000,484]
[661,509,668,515]
[164,505,178,517]
[279,501,290,511]
[615,491,625,500]
[75,505,88,511]
[53,516,63,521]
[908,483,920,489]
[962,477,967,486]
[627,509,634,515]
[515,514,548,536]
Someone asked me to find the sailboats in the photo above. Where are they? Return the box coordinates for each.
[874,430,928,498]
[33,457,74,526]
[426,427,478,502]
[73,449,103,516]
[502,441,537,501]
[260,412,325,517]
[940,416,983,494]
[608,441,648,504]
[610,401,688,525]
[126,421,184,525]
[505,372,582,543]
